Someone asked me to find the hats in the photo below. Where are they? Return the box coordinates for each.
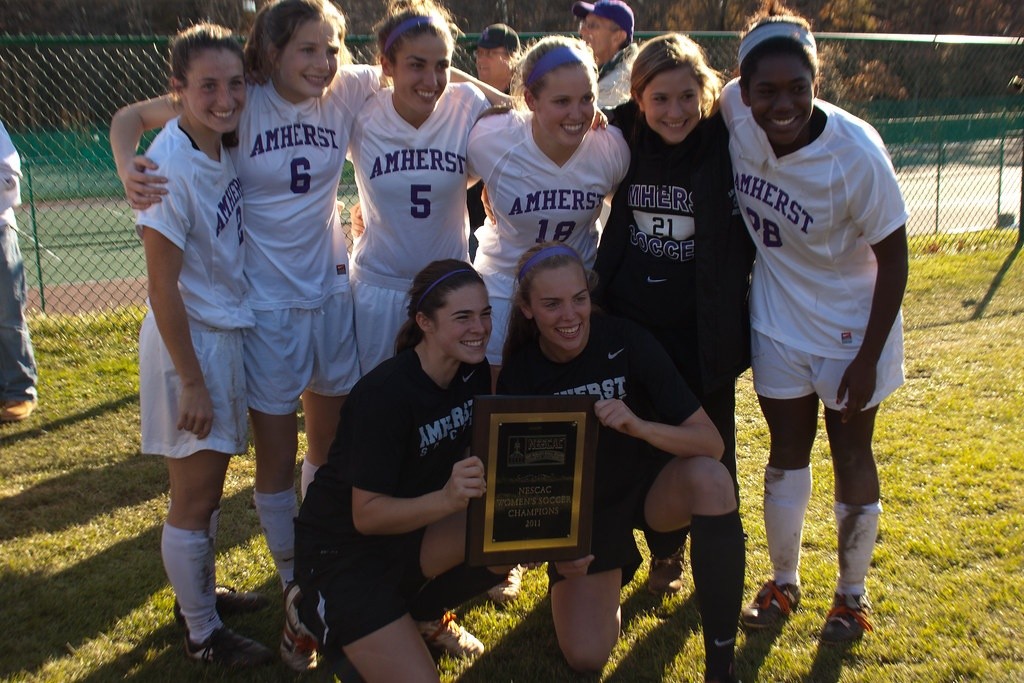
[571,0,634,37]
[463,24,520,54]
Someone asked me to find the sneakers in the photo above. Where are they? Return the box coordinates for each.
[279,582,320,670]
[184,630,275,669]
[647,546,687,594]
[173,586,268,617]
[741,581,802,629]
[417,617,486,658]
[481,566,525,602]
[819,592,871,641]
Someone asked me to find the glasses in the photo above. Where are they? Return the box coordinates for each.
[472,50,503,58]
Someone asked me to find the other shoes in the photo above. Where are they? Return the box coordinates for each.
[1,397,40,422]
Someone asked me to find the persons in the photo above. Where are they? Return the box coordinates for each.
[137,21,275,675]
[503,241,746,683]
[714,1,910,649]
[0,117,38,422]
[282,259,492,683]
[108,0,908,666]
